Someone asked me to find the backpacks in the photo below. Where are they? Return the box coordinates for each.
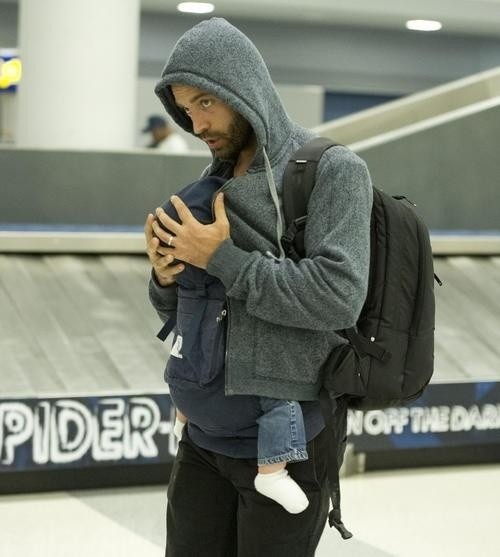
[281,139,436,409]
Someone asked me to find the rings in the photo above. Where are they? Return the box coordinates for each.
[168,236,174,246]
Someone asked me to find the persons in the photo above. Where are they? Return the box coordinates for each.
[143,115,189,154]
[144,17,374,557]
[152,177,311,515]
